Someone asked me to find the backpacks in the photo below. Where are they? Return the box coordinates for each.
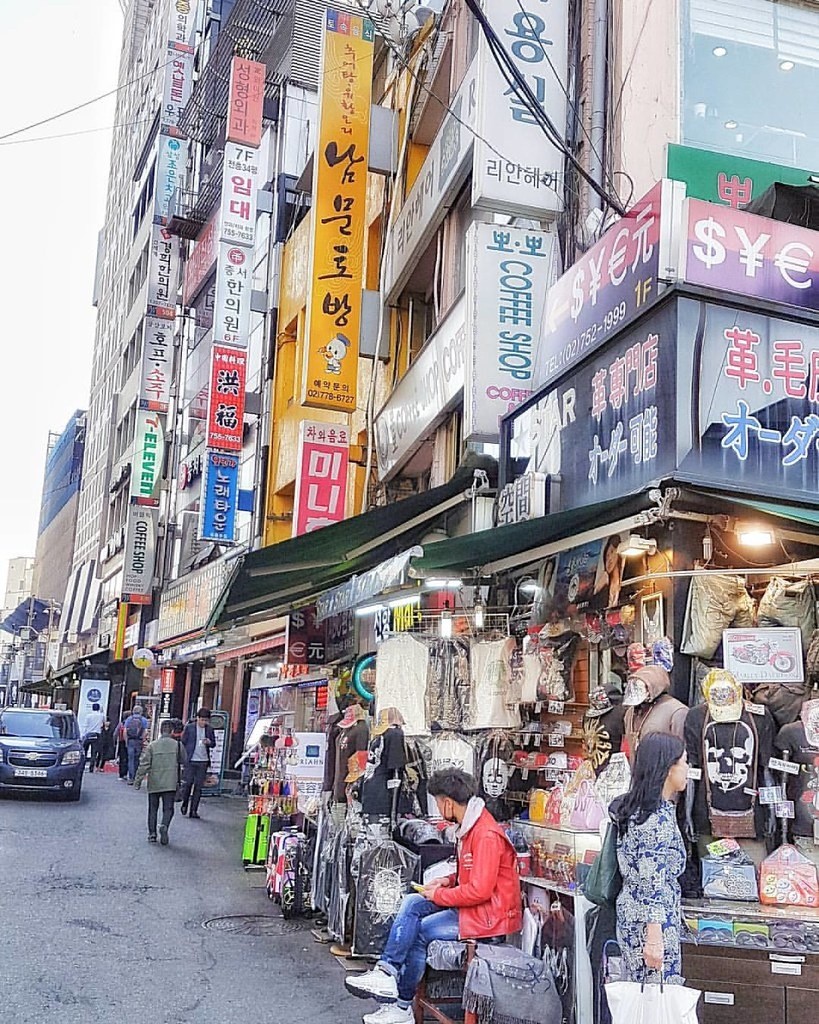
[126,714,143,738]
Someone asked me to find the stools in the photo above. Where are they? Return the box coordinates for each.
[413,936,506,1024]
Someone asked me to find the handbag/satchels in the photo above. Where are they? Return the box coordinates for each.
[544,754,632,829]
[708,805,756,838]
[604,964,702,1024]
[580,822,624,902]
[677,563,819,661]
[175,782,184,803]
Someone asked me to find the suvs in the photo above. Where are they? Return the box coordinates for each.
[0,705,100,803]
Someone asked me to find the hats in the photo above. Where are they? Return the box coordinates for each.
[585,636,673,718]
[507,722,583,770]
[800,699,819,746]
[369,707,405,734]
[336,704,365,729]
[399,817,461,846]
[700,668,743,722]
[345,749,368,784]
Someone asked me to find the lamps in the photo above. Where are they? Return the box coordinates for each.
[733,519,776,547]
[616,534,658,557]
[519,578,536,593]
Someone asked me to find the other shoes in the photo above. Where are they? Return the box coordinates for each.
[159,825,169,845]
[189,809,200,819]
[147,833,156,843]
[181,804,187,816]
[89,767,93,772]
[127,779,133,785]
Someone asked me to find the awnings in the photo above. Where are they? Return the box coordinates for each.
[687,486,819,532]
[315,479,663,625]
[201,469,475,647]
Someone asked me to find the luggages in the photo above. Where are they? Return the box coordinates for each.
[266,825,314,919]
[241,812,271,869]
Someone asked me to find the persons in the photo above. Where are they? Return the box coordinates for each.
[181,708,217,819]
[535,557,556,624]
[124,706,148,785]
[112,711,132,781]
[593,534,623,606]
[82,704,112,772]
[583,666,819,873]
[311,694,407,956]
[134,720,189,845]
[344,766,522,1024]
[615,731,689,986]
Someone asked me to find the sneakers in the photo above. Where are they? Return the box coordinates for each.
[361,1002,416,1024]
[344,965,399,1004]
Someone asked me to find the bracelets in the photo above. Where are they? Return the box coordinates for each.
[646,939,664,945]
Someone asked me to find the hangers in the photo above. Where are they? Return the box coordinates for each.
[551,890,565,922]
[485,626,506,641]
